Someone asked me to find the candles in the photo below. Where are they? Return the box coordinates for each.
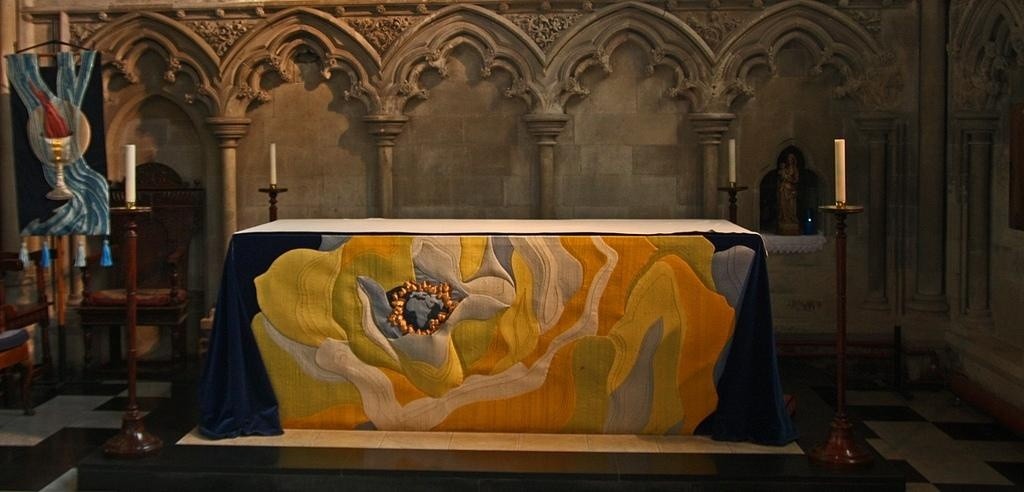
[834,138,847,204]
[269,141,278,186]
[728,136,738,184]
[124,142,138,204]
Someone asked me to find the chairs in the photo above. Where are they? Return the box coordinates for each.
[0,256,56,420]
[77,161,205,394]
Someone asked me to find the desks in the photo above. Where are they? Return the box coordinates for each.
[221,216,769,432]
